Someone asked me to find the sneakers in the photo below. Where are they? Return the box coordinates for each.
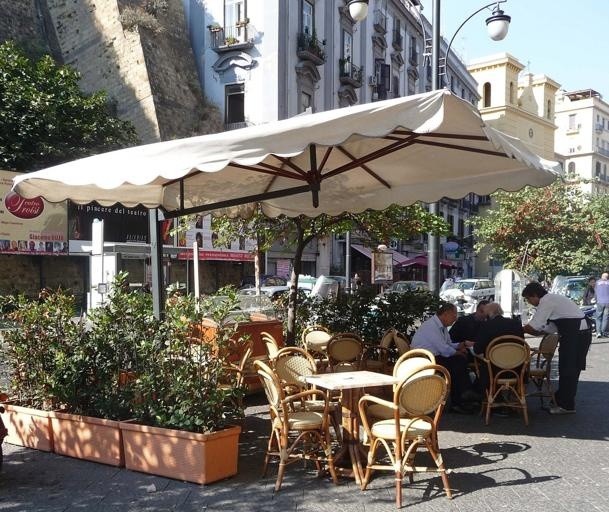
[492,408,509,418]
[550,407,576,415]
[544,400,557,408]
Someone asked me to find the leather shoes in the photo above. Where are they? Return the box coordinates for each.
[450,405,473,415]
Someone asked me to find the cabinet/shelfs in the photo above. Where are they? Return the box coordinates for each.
[168,292,286,400]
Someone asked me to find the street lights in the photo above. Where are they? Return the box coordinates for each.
[337,1,512,314]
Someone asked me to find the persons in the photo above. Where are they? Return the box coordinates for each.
[583,277,597,333]
[410,304,473,415]
[522,281,592,414]
[474,303,528,417]
[594,272,609,338]
[449,301,491,342]
